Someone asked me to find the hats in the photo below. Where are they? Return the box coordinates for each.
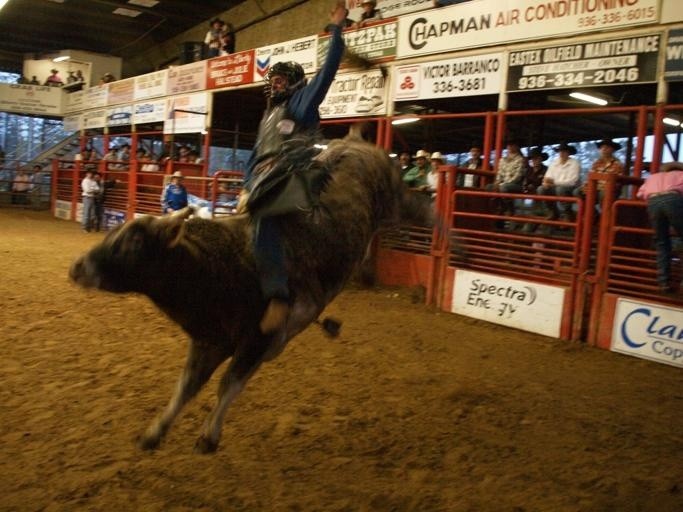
[525,148,550,162]
[51,67,59,74]
[552,142,578,155]
[85,143,192,178]
[399,134,522,169]
[209,17,226,27]
[594,135,623,151]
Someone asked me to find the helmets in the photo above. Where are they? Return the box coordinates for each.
[266,60,310,102]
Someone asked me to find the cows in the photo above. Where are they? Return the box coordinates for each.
[71,122,470,455]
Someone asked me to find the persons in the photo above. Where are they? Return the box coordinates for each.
[29,76,39,85]
[636,162,683,294]
[92,173,105,232]
[238,0,349,335]
[81,170,100,234]
[98,72,116,86]
[17,74,29,84]
[217,23,234,56]
[203,18,224,58]
[76,71,84,81]
[160,171,187,216]
[48,70,62,82]
[71,143,204,172]
[397,137,625,235]
[31,165,43,212]
[67,71,77,84]
[355,0,382,28]
[324,9,356,33]
[12,168,30,210]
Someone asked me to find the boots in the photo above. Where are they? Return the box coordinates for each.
[557,201,576,229]
[544,198,561,224]
